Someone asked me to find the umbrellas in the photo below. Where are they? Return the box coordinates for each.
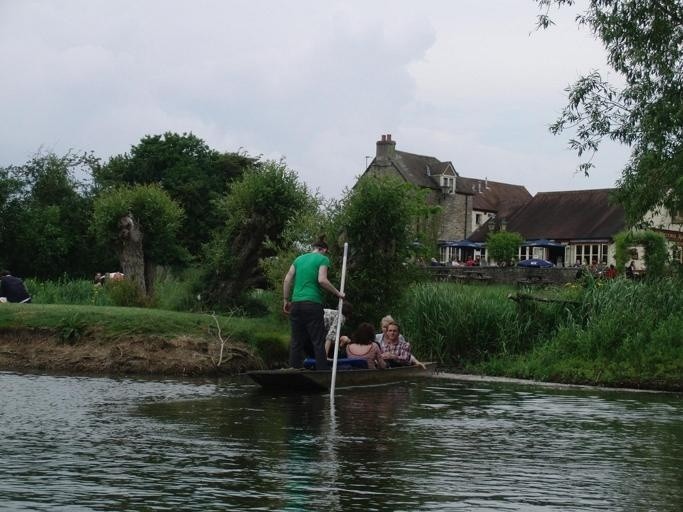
[446,240,482,252]
[436,240,454,248]
[529,239,563,260]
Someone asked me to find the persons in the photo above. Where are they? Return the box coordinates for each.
[302,300,353,363]
[0,270,33,305]
[93,272,103,285]
[280,234,346,369]
[377,322,414,366]
[344,322,388,370]
[373,314,418,364]
[276,379,419,511]
[412,253,638,282]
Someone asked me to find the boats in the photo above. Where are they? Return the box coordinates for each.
[246,358,443,396]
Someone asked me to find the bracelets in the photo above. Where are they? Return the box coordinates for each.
[282,297,291,300]
[334,290,338,296]
